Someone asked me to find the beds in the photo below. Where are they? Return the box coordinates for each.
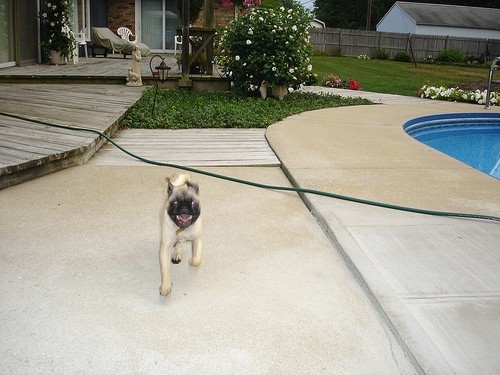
[91,26,153,59]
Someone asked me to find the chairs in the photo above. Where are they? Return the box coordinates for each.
[73,32,89,60]
[117,27,138,44]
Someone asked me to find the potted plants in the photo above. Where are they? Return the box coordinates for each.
[37,1,79,65]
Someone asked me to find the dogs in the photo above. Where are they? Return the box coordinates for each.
[158,173,203,295]
